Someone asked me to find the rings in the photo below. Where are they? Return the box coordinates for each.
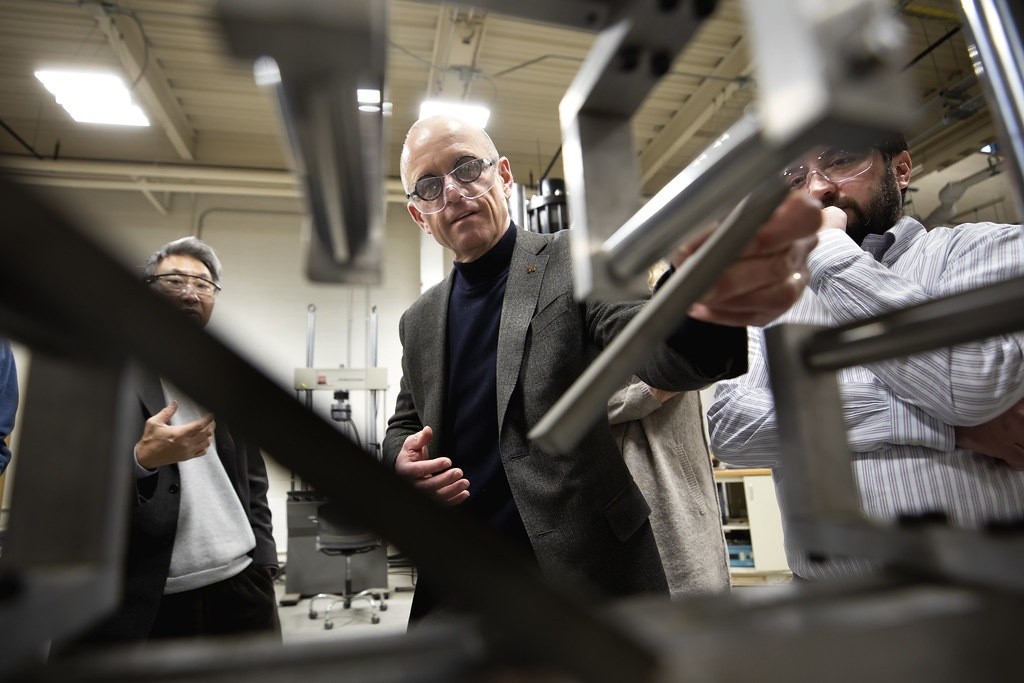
[792,271,806,291]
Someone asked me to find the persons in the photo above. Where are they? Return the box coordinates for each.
[707,110,1024,588]
[608,259,730,594]
[381,116,824,634]
[47,236,281,662]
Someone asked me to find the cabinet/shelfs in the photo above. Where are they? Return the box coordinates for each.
[712,468,793,587]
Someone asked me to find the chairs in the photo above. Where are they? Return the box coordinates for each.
[309,503,388,629]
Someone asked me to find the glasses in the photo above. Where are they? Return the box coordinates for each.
[144,273,222,298]
[406,156,496,201]
[410,156,496,214]
[783,144,880,190]
